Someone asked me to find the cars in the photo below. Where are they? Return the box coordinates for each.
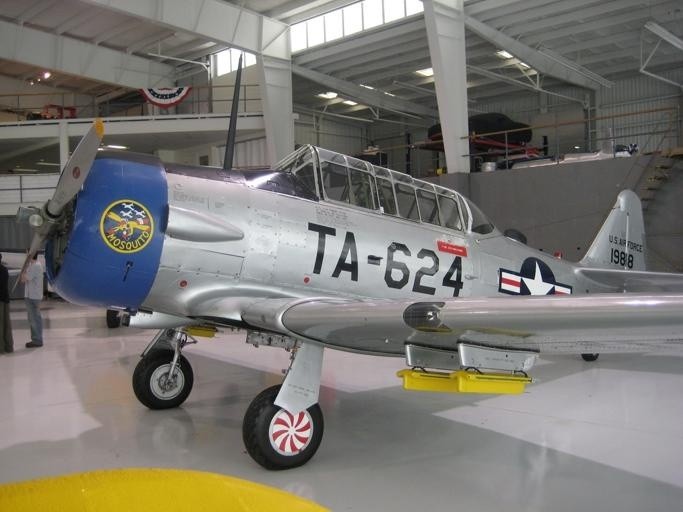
[428,113,533,146]
[511,128,639,169]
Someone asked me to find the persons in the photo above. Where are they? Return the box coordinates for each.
[0,253,14,354]
[24,248,44,348]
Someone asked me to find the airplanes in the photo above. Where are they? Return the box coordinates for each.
[9,51,681,472]
